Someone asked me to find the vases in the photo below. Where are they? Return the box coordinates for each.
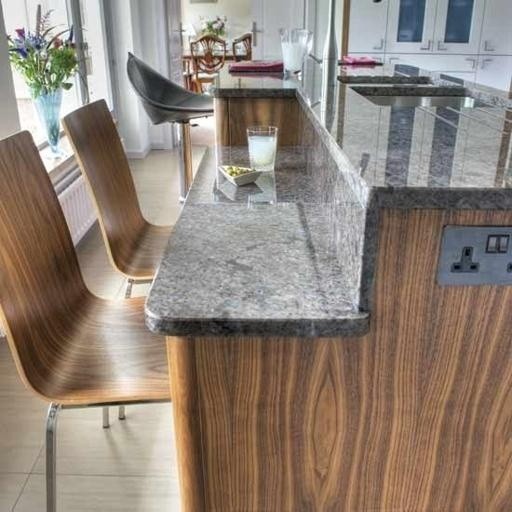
[33,90,66,157]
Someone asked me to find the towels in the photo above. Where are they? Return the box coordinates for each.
[343,55,376,65]
[228,60,283,71]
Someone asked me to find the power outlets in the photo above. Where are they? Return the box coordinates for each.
[435,225,512,286]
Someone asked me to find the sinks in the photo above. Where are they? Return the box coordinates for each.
[335,75,460,87]
[348,84,499,110]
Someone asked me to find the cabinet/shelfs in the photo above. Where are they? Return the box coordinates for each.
[348,0,512,93]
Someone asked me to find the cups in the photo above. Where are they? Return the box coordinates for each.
[246,125,279,172]
[279,28,308,76]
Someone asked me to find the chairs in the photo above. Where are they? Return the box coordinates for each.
[0,130,172,512]
[189,34,226,94]
[62,98,174,420]
[126,51,214,204]
[232,32,252,61]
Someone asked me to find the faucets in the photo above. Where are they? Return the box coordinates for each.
[321,87,371,179]
[322,0,384,87]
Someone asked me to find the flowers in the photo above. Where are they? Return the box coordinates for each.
[198,14,227,38]
[6,4,92,98]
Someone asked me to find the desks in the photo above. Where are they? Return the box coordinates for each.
[182,50,247,93]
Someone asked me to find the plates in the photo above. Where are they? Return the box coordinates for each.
[218,164,263,187]
